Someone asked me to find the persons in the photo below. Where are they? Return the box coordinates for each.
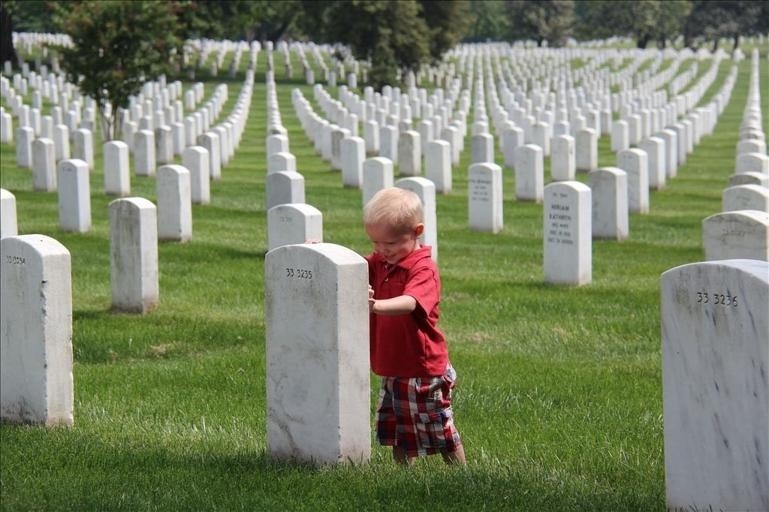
[303,187,465,466]
